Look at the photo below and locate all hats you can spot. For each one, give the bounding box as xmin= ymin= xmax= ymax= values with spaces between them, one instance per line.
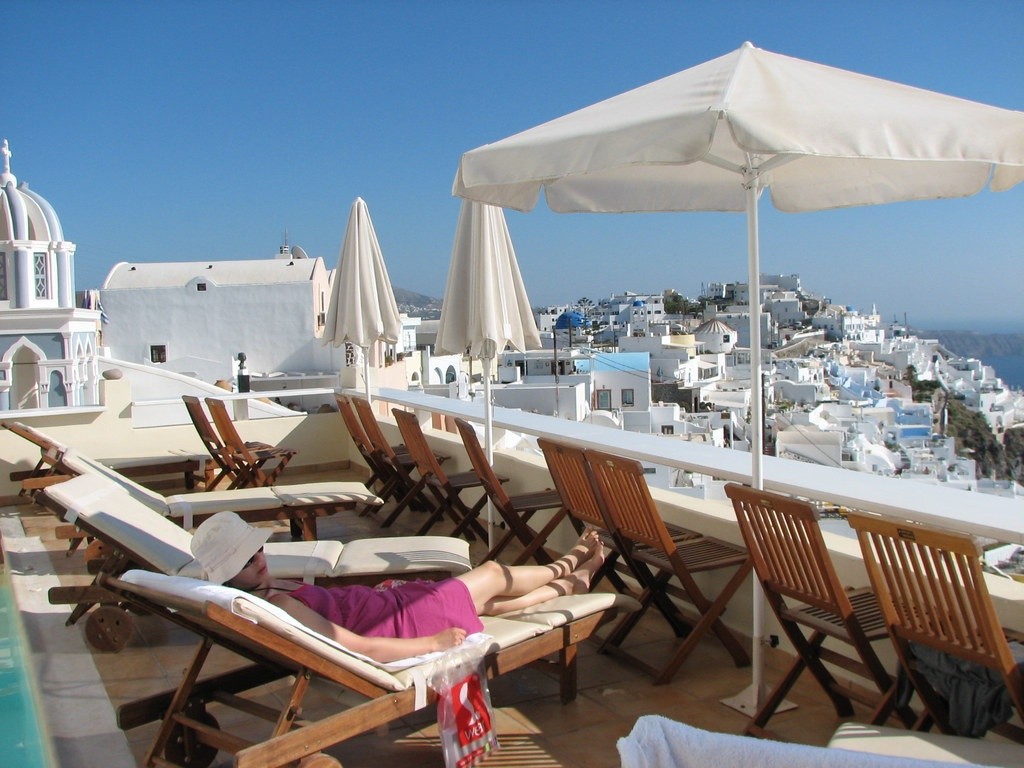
xmin=190 ymin=511 xmax=274 ymax=585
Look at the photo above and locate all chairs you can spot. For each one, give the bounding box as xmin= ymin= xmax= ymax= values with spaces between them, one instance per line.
xmin=0 ymin=387 xmax=1024 ymax=768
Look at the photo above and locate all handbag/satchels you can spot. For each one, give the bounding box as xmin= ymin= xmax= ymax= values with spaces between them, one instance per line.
xmin=423 ymin=646 xmax=500 ymax=768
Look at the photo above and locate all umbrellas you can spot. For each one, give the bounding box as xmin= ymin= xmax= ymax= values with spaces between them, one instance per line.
xmin=450 ymin=40 xmax=1024 ymax=692
xmin=320 ymin=196 xmax=403 ymax=483
xmin=434 ymin=194 xmax=543 ymax=553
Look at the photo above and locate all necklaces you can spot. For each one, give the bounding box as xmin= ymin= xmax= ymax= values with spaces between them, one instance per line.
xmin=251 ymin=585 xmax=302 ymax=597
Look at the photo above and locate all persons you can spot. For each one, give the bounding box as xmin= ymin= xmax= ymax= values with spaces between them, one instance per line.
xmin=187 ymin=510 xmax=608 ymax=664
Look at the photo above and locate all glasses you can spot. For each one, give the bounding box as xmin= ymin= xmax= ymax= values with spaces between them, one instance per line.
xmin=242 ymin=545 xmax=263 ymax=570
